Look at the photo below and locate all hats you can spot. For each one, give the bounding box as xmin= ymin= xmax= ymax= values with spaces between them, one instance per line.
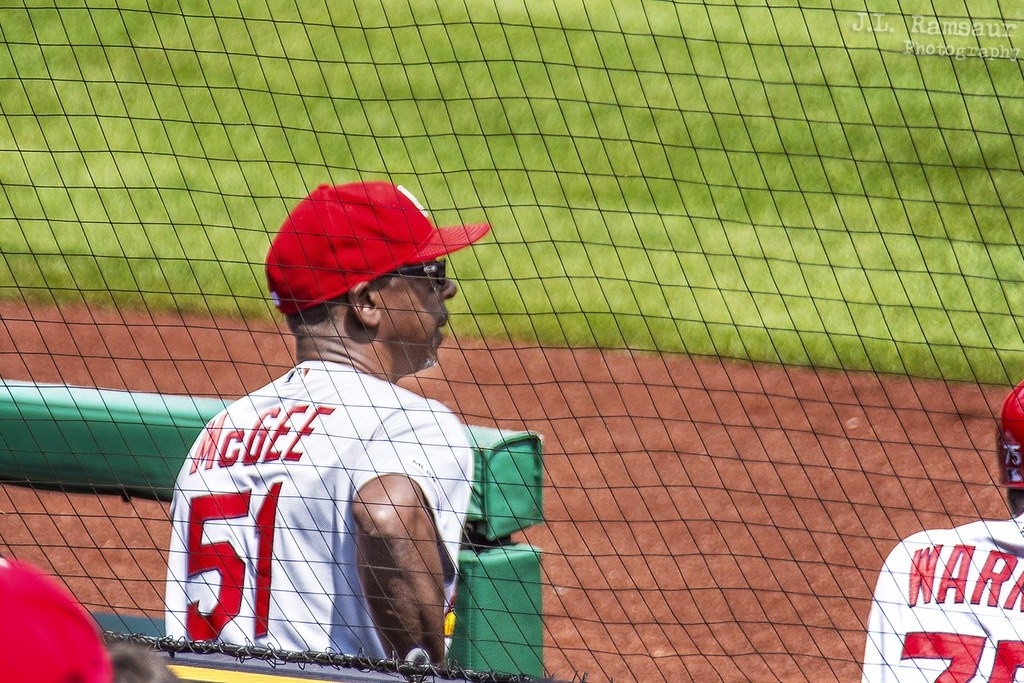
xmin=265 ymin=182 xmax=491 ymax=315
xmin=0 ymin=554 xmax=119 ymax=683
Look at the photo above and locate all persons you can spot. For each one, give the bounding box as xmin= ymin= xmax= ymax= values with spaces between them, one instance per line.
xmin=858 ymin=378 xmax=1024 ymax=683
xmin=162 ymin=182 xmax=476 ymax=672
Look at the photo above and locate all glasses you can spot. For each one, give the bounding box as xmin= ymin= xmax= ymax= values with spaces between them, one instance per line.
xmin=391 ymin=258 xmax=446 ymax=286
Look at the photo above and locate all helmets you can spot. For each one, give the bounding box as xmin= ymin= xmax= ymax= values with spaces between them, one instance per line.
xmin=995 ymin=380 xmax=1024 ymax=488
